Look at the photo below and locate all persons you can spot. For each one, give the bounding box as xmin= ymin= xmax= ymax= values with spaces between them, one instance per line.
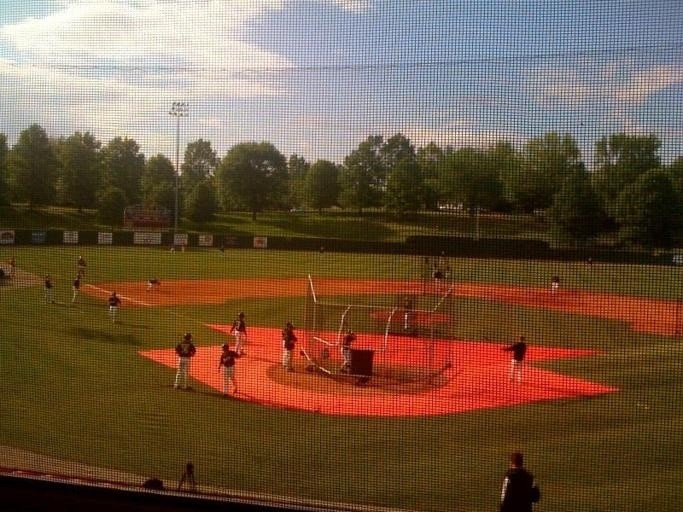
xmin=171 ymin=331 xmax=196 ymax=390
xmin=9 ymin=253 xmax=16 ymax=278
xmin=548 ymin=270 xmax=560 ymax=296
xmin=403 ymin=295 xmax=414 ymax=330
xmin=43 ymin=272 xmax=54 ymax=304
xmin=76 ymin=254 xmax=86 ymax=277
xmin=339 ymin=330 xmax=356 ymax=370
xmin=226 ymin=311 xmax=248 ymax=357
xmin=280 ymin=322 xmax=296 ymax=373
xmin=145 ymin=278 xmax=160 ymax=294
xmin=216 ymin=342 xmax=242 ymax=397
xmin=70 ymin=271 xmax=81 ymax=305
xmin=503 ymin=335 xmax=524 ymax=386
xmin=105 ymin=291 xmax=121 ymax=326
xmin=497 ymin=451 xmax=539 ymax=512
xmin=430 ymin=263 xmax=444 ymax=293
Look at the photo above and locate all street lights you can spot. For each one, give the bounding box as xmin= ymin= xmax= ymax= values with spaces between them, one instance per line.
xmin=169 ymin=101 xmax=190 ymax=228
